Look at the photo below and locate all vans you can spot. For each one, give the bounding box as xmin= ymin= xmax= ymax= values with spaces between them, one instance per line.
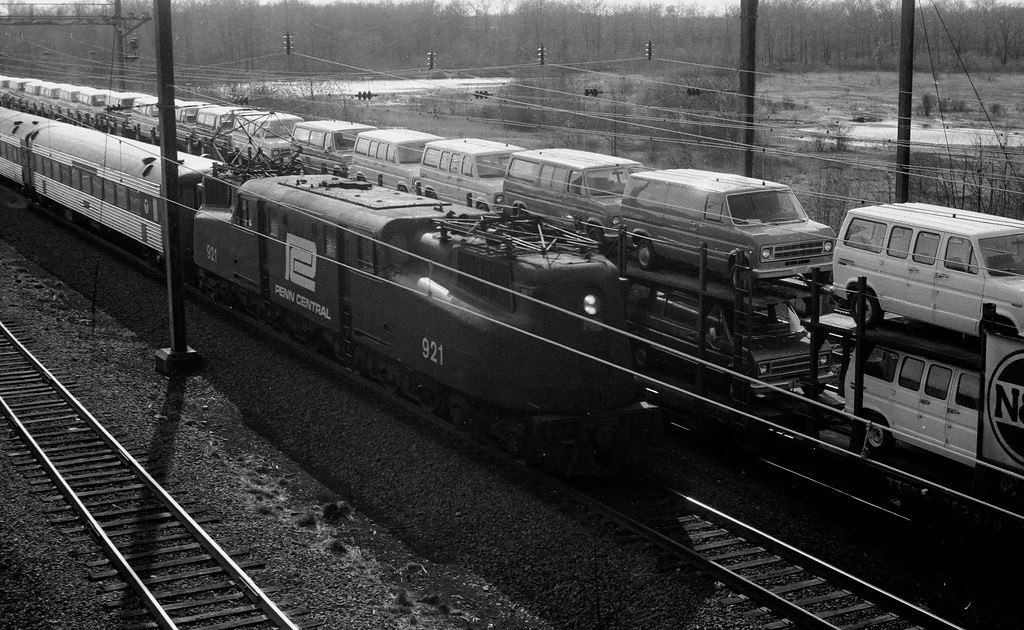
xmin=419 ymin=138 xmax=528 ymax=215
xmin=841 ymin=342 xmax=981 ymax=471
xmin=830 ymin=202 xmax=1024 ymax=343
xmin=193 ymin=105 xmax=252 ymax=156
xmin=618 ymin=168 xmax=837 ymax=298
xmin=130 ymin=97 xmax=184 ymax=145
xmin=230 ymin=110 xmax=305 ymax=169
xmin=289 ymin=118 xmax=379 ymax=177
xmin=0 ymin=75 xmax=155 ymax=137
xmin=349 ymin=127 xmax=446 ymax=196
xmin=174 ymin=100 xmax=213 ymax=154
xmin=502 ymin=148 xmax=647 ymax=250
xmin=623 ymin=280 xmax=835 ymax=402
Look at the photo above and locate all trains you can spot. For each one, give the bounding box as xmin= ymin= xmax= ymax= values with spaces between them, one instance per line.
xmin=0 ymin=106 xmax=664 ymax=484
